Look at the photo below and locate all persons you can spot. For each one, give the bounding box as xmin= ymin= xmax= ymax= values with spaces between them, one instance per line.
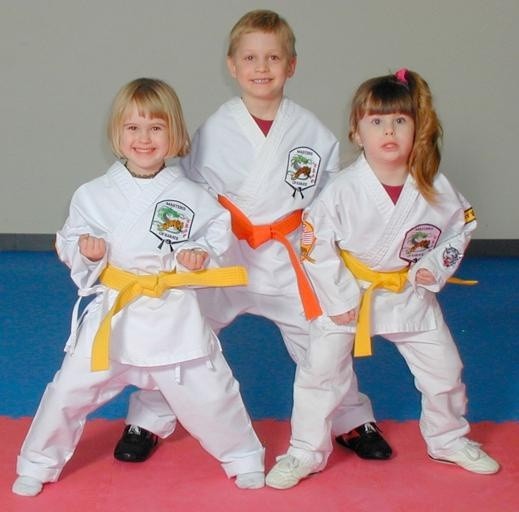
xmin=113 ymin=8 xmax=393 ymax=461
xmin=12 ymin=77 xmax=265 ymax=497
xmin=264 ymin=67 xmax=501 ymax=491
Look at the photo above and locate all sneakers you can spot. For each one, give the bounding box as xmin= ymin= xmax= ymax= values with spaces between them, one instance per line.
xmin=112 ymin=424 xmax=160 ymax=462
xmin=265 ymin=456 xmax=319 ymax=490
xmin=428 ymin=439 xmax=501 ymax=475
xmin=333 ymin=422 xmax=393 ymax=459
